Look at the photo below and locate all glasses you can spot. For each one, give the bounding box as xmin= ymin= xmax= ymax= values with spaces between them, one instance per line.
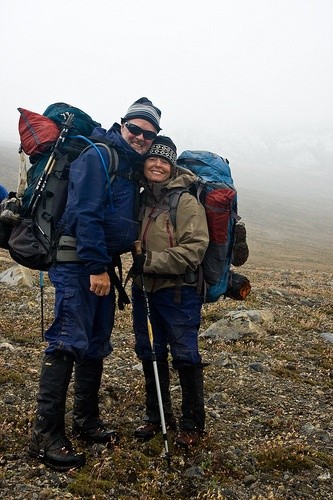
xmin=124 ymin=120 xmax=157 ymax=141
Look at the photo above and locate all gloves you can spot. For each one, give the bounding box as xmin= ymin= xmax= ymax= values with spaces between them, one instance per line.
xmin=134 ymin=254 xmax=146 ymax=267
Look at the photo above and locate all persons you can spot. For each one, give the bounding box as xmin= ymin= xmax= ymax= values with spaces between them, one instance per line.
xmin=133 ymin=135 xmax=210 ymax=446
xmin=26 ymin=97 xmax=161 ymax=471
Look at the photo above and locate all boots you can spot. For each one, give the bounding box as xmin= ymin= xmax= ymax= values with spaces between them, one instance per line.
xmin=174 ymin=363 xmax=204 ymax=447
xmin=71 ymin=356 xmax=120 ymax=445
xmin=133 ymin=361 xmax=174 ymax=438
xmin=27 ymin=354 xmax=85 ymax=471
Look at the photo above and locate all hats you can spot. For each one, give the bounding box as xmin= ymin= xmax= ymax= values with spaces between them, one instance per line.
xmin=124 ymin=96 xmax=161 ymax=131
xmin=143 ymin=135 xmax=177 ymax=169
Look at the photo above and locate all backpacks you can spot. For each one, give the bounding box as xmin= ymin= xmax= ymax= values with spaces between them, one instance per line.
xmin=136 ymin=150 xmax=251 ymax=303
xmin=0 ymin=103 xmax=117 ymax=270
